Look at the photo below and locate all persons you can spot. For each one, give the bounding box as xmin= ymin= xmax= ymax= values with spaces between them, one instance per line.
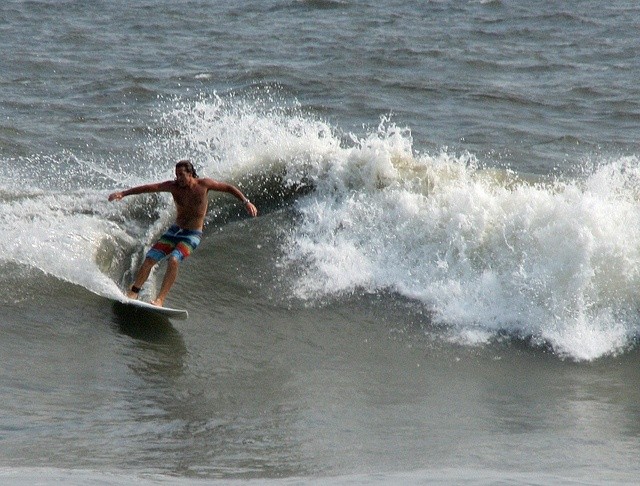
xmin=109 ymin=161 xmax=258 ymax=306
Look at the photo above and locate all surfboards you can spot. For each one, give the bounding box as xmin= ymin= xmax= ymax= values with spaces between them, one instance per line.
xmin=123 ymin=266 xmax=188 ymax=316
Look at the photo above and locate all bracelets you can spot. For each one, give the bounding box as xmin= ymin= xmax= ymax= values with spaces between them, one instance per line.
xmin=243 ymin=199 xmax=250 ymax=205
